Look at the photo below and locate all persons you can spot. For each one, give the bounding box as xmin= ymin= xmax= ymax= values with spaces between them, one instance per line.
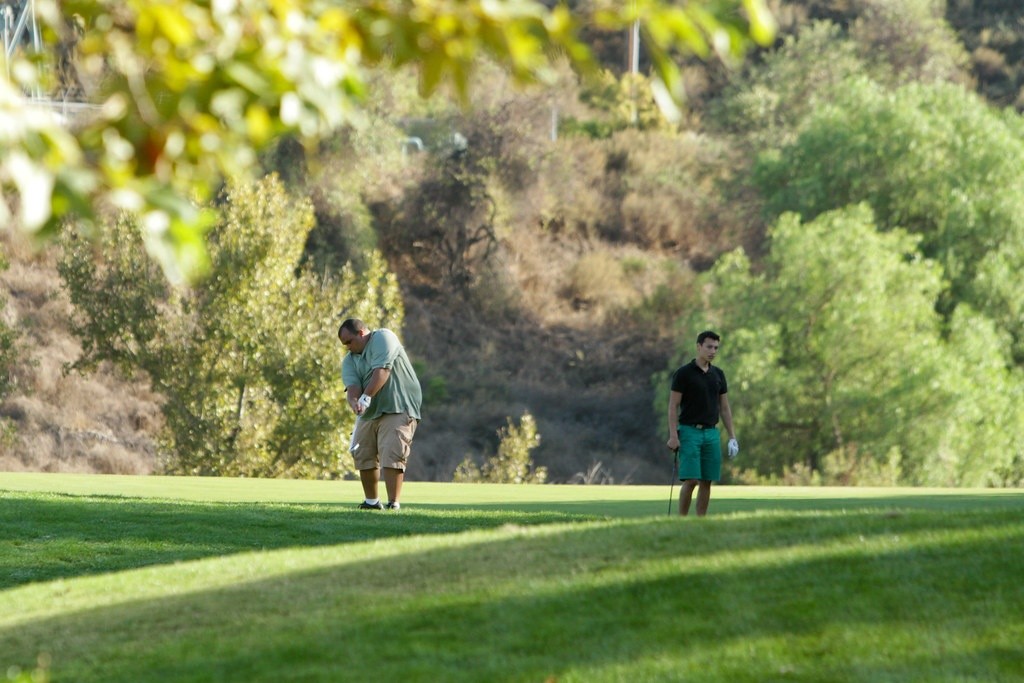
xmin=668 ymin=331 xmax=738 ymax=516
xmin=338 ymin=318 xmax=423 ymax=510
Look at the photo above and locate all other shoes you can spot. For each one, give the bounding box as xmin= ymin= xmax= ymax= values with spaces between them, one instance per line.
xmin=385 ymin=503 xmax=400 ymax=509
xmin=357 ymin=501 xmax=383 ymax=510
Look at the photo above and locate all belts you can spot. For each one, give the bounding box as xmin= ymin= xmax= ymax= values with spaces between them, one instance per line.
xmin=680 ymin=422 xmax=715 ymax=429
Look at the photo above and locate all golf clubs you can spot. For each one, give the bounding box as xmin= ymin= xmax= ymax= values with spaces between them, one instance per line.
xmin=348 ymin=413 xmax=360 ymax=452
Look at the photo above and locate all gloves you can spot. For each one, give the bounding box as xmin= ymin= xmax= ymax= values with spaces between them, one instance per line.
xmin=728 ymin=439 xmax=738 ymax=459
xmin=358 ymin=394 xmax=371 ymax=416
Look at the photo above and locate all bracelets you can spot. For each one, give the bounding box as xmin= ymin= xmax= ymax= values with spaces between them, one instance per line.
xmin=359 ymin=394 xmax=371 ymax=408
